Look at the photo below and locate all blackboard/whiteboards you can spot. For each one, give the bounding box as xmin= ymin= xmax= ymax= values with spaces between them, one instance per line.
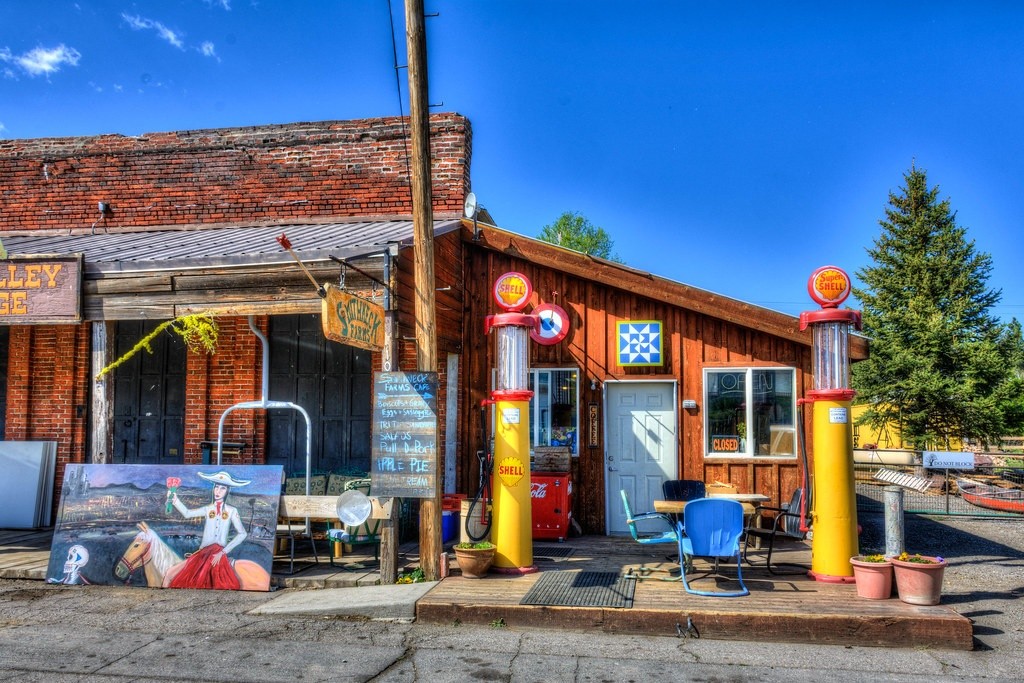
xmin=368 ymin=367 xmax=440 ymax=499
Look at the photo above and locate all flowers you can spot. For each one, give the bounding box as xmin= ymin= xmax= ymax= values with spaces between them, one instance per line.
xmin=860 ymin=555 xmax=886 ymax=563
xmin=898 ymin=552 xmax=944 ymax=564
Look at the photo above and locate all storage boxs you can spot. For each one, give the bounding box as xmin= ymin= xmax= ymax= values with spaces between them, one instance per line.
xmin=704 ymin=483 xmax=740 ymax=494
xmin=418 ymin=510 xmax=459 ymax=544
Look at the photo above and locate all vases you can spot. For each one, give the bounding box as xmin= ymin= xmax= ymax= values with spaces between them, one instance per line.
xmin=890 ymin=555 xmax=947 ymax=606
xmin=850 ymin=556 xmax=894 ymax=599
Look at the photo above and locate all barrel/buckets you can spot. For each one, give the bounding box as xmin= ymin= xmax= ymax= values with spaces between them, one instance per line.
xmin=460 ymin=498 xmax=493 ymax=543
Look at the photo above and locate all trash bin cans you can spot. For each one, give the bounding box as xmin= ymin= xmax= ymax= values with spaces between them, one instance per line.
xmin=460 ymin=497 xmax=491 ymax=544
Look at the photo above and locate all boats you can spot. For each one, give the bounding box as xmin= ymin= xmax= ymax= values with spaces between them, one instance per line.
xmin=955 ymin=479 xmax=1024 ymax=515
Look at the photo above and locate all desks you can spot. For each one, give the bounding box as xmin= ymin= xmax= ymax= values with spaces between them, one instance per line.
xmin=653 ymin=500 xmax=756 ymax=584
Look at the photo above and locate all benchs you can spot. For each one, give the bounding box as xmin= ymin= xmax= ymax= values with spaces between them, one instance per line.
xmin=284 ymin=465 xmax=408 ymax=547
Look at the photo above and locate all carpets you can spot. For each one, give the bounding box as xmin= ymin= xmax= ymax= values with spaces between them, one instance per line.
xmin=517 ymin=569 xmax=638 ymax=609
xmin=532 ymin=546 xmax=576 ymax=568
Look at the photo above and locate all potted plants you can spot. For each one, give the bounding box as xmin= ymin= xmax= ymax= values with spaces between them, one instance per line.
xmin=736 ymin=422 xmax=756 ymax=452
xmin=452 ymin=541 xmax=497 ymax=579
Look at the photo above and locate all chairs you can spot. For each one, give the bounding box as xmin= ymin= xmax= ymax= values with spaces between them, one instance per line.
xmin=620 ymin=489 xmax=690 ymax=582
xmin=662 ymin=480 xmax=729 ymax=564
xmin=272 ymin=495 xmax=319 ymax=575
xmin=326 ymin=479 xmax=382 ymax=572
xmin=677 ymin=498 xmax=750 ymax=598
xmin=744 ymin=487 xmax=813 ymax=577
